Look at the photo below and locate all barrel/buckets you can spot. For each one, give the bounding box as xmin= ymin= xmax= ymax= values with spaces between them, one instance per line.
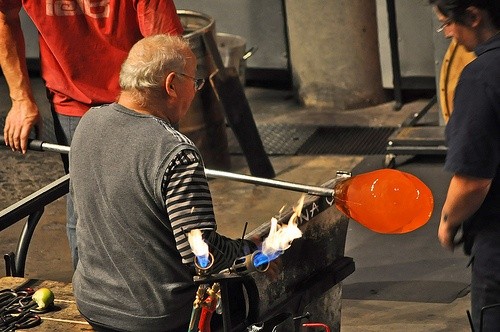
xmin=218 ymin=34 xmax=259 ymax=125
xmin=176 ymin=10 xmax=231 ymax=181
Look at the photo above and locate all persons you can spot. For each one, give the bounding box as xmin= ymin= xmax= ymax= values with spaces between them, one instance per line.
xmin=429 ymin=0 xmax=500 ymax=332
xmin=0 ymin=0 xmax=186 ymax=271
xmin=69 ymin=34 xmax=263 ymax=332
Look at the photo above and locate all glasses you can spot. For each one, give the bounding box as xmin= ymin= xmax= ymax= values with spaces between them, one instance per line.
xmin=181 ymin=73 xmax=205 ymax=90
xmin=432 ymin=17 xmax=453 ymax=36
